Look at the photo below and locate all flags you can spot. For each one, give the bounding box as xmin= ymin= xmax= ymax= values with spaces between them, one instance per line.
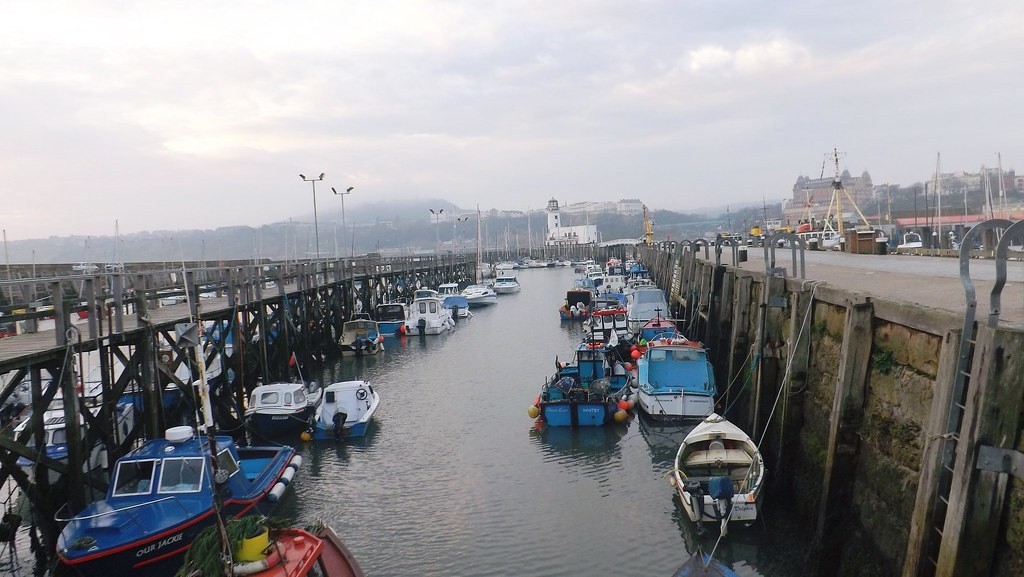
xmin=289 ymin=355 xmax=295 ymax=366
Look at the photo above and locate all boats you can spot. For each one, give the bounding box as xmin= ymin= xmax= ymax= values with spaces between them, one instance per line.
xmin=1 ymin=257 xmax=765 ymax=577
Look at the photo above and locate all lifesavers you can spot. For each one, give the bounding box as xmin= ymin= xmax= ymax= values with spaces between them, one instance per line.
xmin=586 ymin=342 xmax=604 ymax=349
xmin=225 ymin=539 xmax=285 ymax=575
